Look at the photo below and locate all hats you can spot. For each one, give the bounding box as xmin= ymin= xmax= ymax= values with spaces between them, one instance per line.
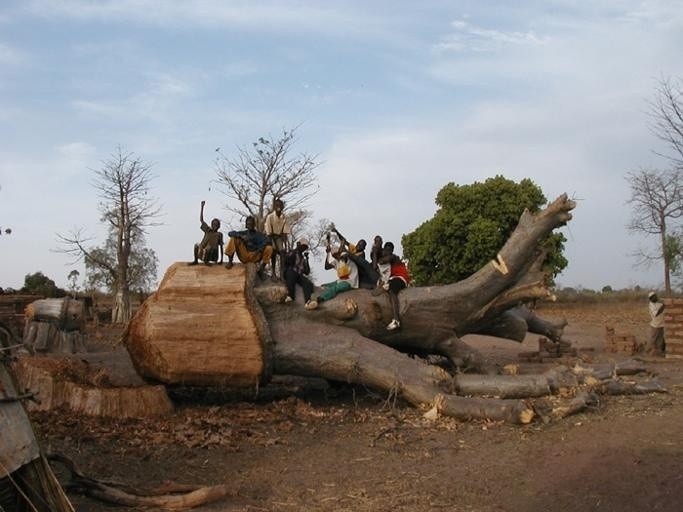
xmin=300 ymin=238 xmax=309 ymax=248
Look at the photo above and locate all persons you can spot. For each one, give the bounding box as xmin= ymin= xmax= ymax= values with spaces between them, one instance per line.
xmin=223 ymin=215 xmax=273 ymax=281
xmin=281 ymin=237 xmax=318 ymax=310
xmin=641 ymin=290 xmax=667 ymax=359
xmin=263 ymin=199 xmax=289 ymax=282
xmin=313 ymin=221 xmax=410 ymax=330
xmin=186 ymin=200 xmax=224 ymax=267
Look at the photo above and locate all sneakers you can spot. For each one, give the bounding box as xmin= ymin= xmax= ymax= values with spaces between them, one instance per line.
xmin=339 ymin=239 xmax=346 ymax=253
xmin=304 ymin=296 xmax=322 ymax=310
xmin=285 ymin=296 xmax=292 ymax=303
xmin=386 ymin=318 xmax=400 ymax=330
xmin=382 ymin=283 xmax=392 ymax=291
